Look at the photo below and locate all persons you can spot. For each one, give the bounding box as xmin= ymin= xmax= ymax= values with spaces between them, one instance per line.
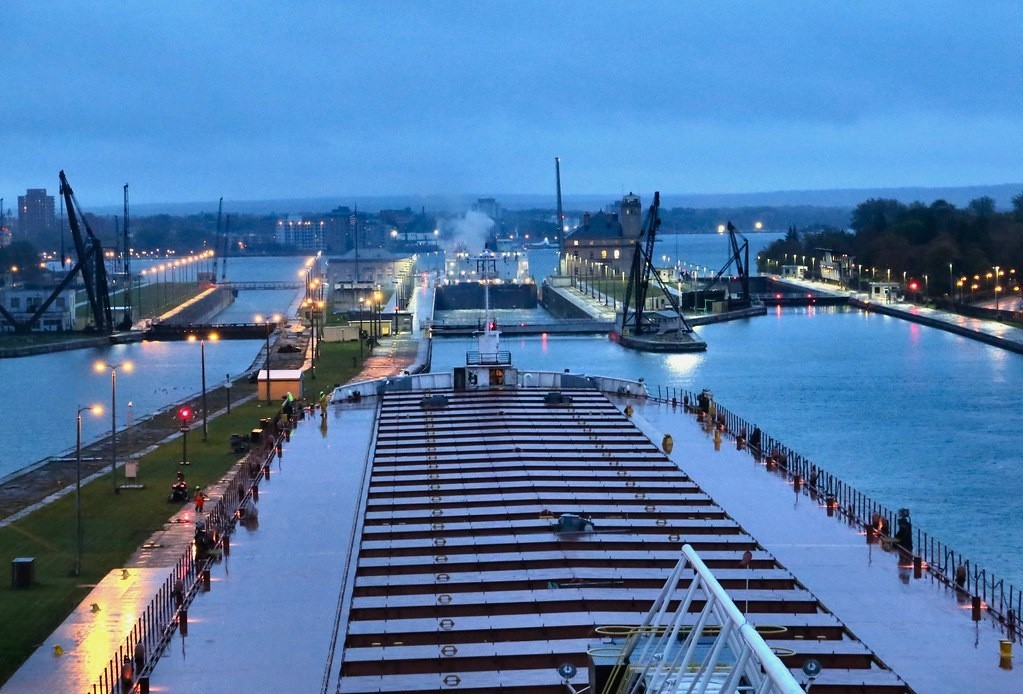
xmin=262 ymin=430 xmax=274 ymax=451
xmin=281 ymin=395 xmax=292 ymax=419
xmin=237 ymin=483 xmax=246 ymax=502
xmin=251 ymin=455 xmax=260 ymax=477
xmin=195 ymin=486 xmax=209 ymax=512
xmin=319 ymin=391 xmax=327 ymax=416
xmin=176 ymin=471 xmax=184 ymax=481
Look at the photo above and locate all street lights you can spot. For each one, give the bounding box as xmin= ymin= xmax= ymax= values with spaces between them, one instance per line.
xmin=857 ymin=264 xmax=863 ymax=292
xmin=358 ymin=297 xmax=365 ymax=357
xmin=921 ymin=274 xmax=929 ymax=307
xmin=136 ymin=248 xmax=216 ymax=324
xmin=297 ymin=255 xmax=327 ymax=379
xmin=366 ymin=299 xmax=374 ymax=351
xmin=254 ymin=313 xmax=282 ymax=405
xmin=872 ymin=267 xmax=876 ymax=282
xmin=375 ymin=293 xmax=384 ymax=338
xmin=886 ymin=268 xmax=892 ymax=305
xmin=188 ymin=332 xmax=220 ymax=442
xmin=811 ymin=257 xmax=815 ymax=280
xmin=76 ymin=406 xmax=104 ymax=575
xmin=802 ymin=256 xmax=805 ymax=280
xmin=960 ymin=276 xmax=967 ymax=305
xmin=992 ymin=265 xmax=1000 ymax=310
xmin=94 ymin=360 xmax=135 ymax=493
xmin=784 ymin=253 xmax=787 ymax=277
xmin=793 ymin=254 xmax=797 ymax=279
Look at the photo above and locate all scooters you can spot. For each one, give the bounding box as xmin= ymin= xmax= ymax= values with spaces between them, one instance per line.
xmin=168 ymin=482 xmax=190 ymax=502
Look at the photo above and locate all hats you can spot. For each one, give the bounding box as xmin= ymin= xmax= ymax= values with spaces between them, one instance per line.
xmin=196 ymin=486 xmax=200 ymax=489
xmin=320 ymin=391 xmax=323 ymax=395
xmin=287 ymin=392 xmax=290 ymax=394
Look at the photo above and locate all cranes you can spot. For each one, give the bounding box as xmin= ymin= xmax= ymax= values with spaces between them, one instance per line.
xmin=617 ymin=190 xmax=694 ymax=336
xmin=700 ymin=219 xmax=752 ymax=312
xmin=115 ymin=182 xmax=134 ymax=327
xmin=211 ymin=194 xmax=230 ymax=287
xmin=55 ymin=168 xmax=115 ymax=334
xmin=552 ymin=156 xmax=566 ymax=275
